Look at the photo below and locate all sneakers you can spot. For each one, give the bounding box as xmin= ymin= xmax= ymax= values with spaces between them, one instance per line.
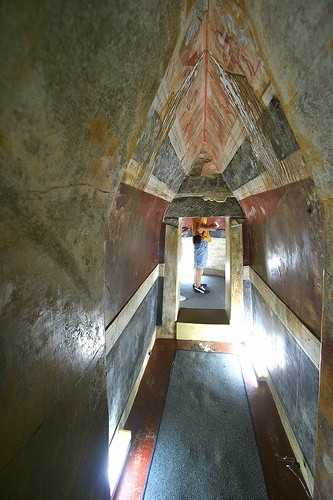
xmin=194 ymin=286 xmax=210 ymax=294
xmin=193 ymin=284 xmax=207 ymax=289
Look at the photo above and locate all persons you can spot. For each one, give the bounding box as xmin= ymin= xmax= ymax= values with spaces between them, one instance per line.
xmin=191 ymin=217 xmax=218 ymax=295
